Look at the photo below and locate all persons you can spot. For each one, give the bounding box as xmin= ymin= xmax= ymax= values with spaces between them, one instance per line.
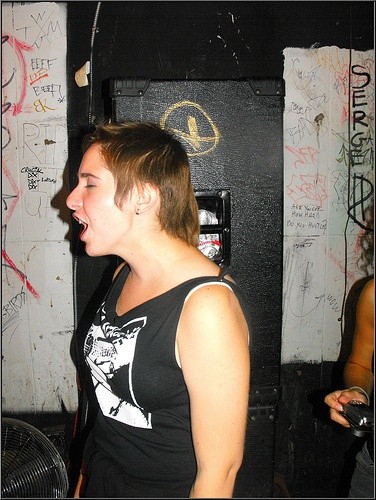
xmin=66 ymin=123 xmax=249 ymax=499
xmin=325 ymin=279 xmax=375 ymax=498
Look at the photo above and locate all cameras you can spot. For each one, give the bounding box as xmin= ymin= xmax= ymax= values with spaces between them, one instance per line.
xmin=338 ymin=400 xmax=374 ymax=437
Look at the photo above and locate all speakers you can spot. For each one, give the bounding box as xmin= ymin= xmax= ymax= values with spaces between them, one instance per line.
xmin=105 ymin=77 xmax=285 ymax=500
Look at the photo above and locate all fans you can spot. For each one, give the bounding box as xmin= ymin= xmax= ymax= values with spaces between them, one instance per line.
xmin=2 ymin=417 xmax=69 ymax=498
